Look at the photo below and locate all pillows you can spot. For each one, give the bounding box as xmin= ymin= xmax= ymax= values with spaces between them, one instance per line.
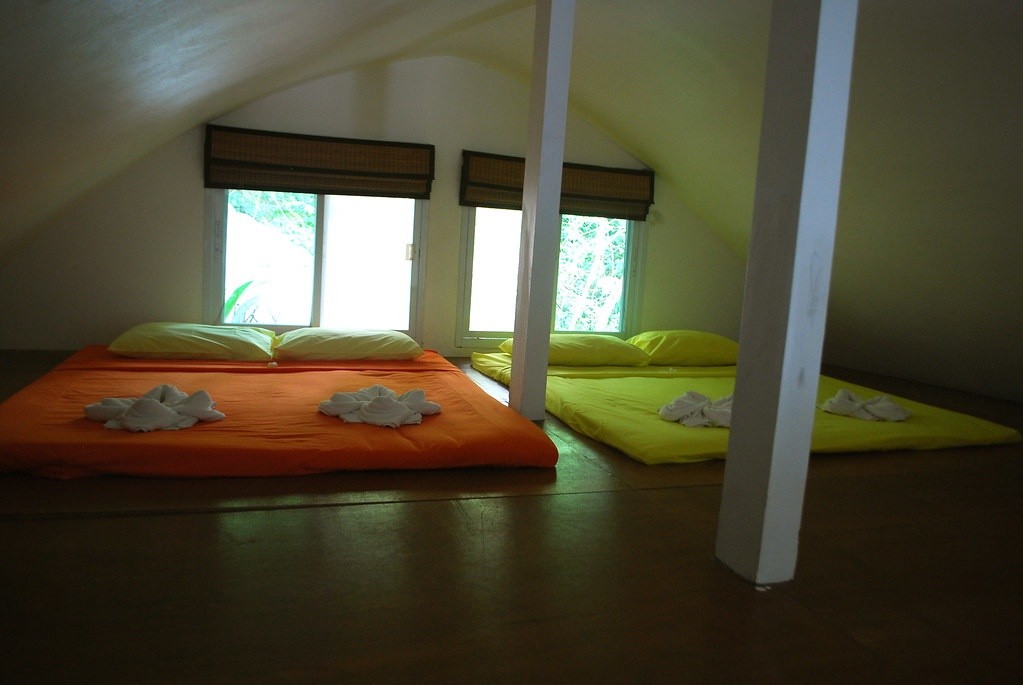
xmin=626 ymin=330 xmax=740 ymax=367
xmin=274 ymin=328 xmax=426 ymax=362
xmin=106 ymin=321 xmax=275 ymax=363
xmin=502 ymin=332 xmax=653 ymax=368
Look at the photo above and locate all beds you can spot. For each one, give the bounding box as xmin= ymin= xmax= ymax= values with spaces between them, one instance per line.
xmin=0 ymin=341 xmax=559 ymax=480
xmin=471 ymin=330 xmax=1023 ymax=465
